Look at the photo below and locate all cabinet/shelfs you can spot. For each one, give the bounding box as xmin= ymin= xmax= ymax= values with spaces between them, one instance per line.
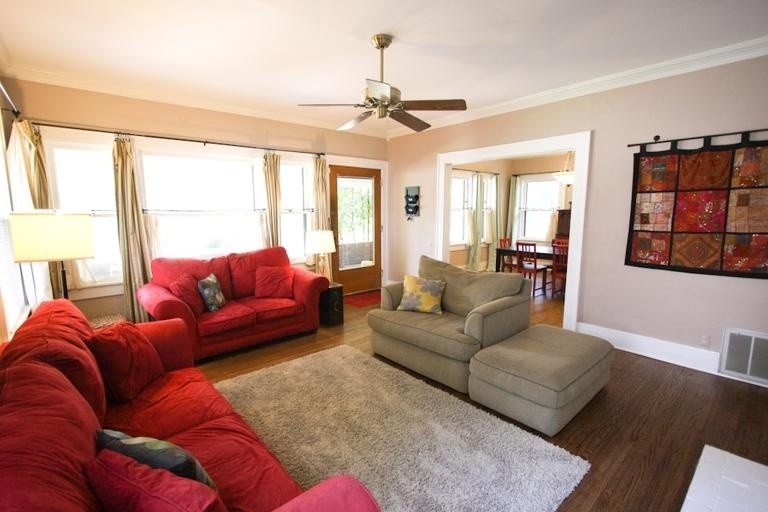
xmin=556 ymin=210 xmax=570 ymax=240
xmin=320 ymin=282 xmax=344 ymax=328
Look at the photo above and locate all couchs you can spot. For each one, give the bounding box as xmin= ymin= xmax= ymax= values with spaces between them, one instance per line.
xmin=0 ymin=297 xmax=382 ymax=512
xmin=136 ymin=247 xmax=329 ymax=366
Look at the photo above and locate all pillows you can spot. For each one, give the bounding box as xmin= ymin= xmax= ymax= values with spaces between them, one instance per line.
xmin=254 ymin=266 xmax=294 ymax=297
xmin=395 ymin=275 xmax=447 ymax=314
xmin=95 ymin=429 xmax=218 ymax=492
xmin=83 ymin=321 xmax=164 ymax=404
xmin=86 ymin=448 xmax=228 ymax=512
xmin=197 ymin=272 xmax=226 ymax=312
xmin=169 ymin=273 xmax=208 ymax=318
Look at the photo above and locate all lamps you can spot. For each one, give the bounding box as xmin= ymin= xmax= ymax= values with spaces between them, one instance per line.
xmin=9 ymin=210 xmax=94 ymax=298
xmin=305 ymin=230 xmax=336 ymax=284
xmin=552 ymin=151 xmax=574 ymax=187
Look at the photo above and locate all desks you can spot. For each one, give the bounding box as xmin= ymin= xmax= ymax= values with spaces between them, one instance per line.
xmin=90 ymin=312 xmax=127 ymax=326
xmin=496 ymin=245 xmax=568 ymax=272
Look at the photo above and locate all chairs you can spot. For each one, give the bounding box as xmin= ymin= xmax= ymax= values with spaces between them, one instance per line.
xmin=366 ymin=253 xmax=532 ymax=395
xmin=543 ymin=240 xmax=569 ymax=296
xmin=551 ymin=239 xmax=569 ymax=299
xmin=499 ymin=238 xmax=517 ymax=273
xmin=514 ymin=242 xmax=548 ymax=301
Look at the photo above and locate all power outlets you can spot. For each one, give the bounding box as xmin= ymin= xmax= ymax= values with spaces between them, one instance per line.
xmin=700 ymin=334 xmax=709 ymax=346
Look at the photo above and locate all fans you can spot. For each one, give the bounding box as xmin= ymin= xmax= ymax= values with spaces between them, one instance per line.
xmin=297 ymin=32 xmax=467 ymax=133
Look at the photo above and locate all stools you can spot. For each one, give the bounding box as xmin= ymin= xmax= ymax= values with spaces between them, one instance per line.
xmin=468 ymin=323 xmax=615 ymax=437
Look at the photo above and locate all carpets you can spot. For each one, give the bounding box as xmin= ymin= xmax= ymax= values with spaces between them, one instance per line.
xmin=213 ymin=344 xmax=592 ymax=511
